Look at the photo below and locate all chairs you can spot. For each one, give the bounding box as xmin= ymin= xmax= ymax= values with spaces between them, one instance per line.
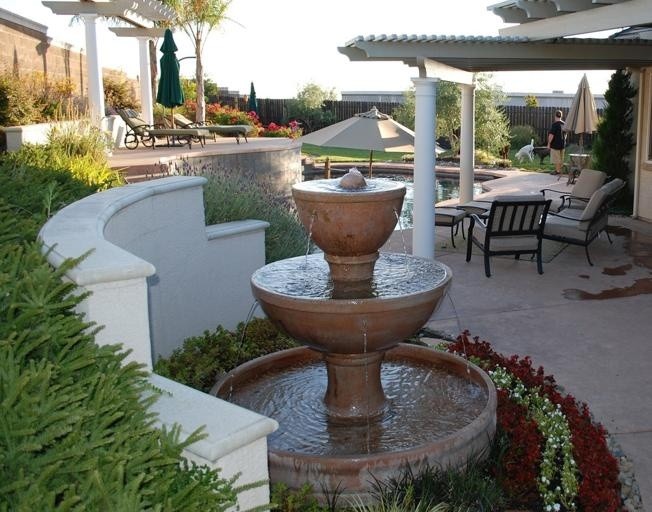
xmin=466 ymin=168 xmax=627 ymax=279
xmin=112 ymin=105 xmax=252 ymax=149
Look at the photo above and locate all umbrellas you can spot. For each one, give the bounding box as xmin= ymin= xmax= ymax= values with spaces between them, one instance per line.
xmin=156 ymin=28 xmax=185 ymax=145
xmin=562 ymin=73 xmax=598 ymax=154
xmin=300 ymin=106 xmax=447 ymax=178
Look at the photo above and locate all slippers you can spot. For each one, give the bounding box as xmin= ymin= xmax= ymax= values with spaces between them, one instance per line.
xmin=550 ymin=170 xmax=561 ymax=175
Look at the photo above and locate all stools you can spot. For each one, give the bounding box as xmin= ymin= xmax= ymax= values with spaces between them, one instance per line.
xmin=557 ymin=162 xmax=572 ymax=181
xmin=455 ymin=202 xmax=492 ymax=240
xmin=435 ymin=207 xmax=467 ymax=248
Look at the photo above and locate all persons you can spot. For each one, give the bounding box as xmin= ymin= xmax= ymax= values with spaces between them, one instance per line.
xmin=547 ymin=110 xmax=567 ymax=177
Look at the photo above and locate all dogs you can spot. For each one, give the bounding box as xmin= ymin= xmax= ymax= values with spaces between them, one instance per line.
xmin=515 ymin=138 xmax=534 ymax=165
xmin=533 ymin=147 xmax=550 ymax=164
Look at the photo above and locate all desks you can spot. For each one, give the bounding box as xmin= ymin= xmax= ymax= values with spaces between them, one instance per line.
xmin=567 ymin=153 xmax=591 ymax=186
